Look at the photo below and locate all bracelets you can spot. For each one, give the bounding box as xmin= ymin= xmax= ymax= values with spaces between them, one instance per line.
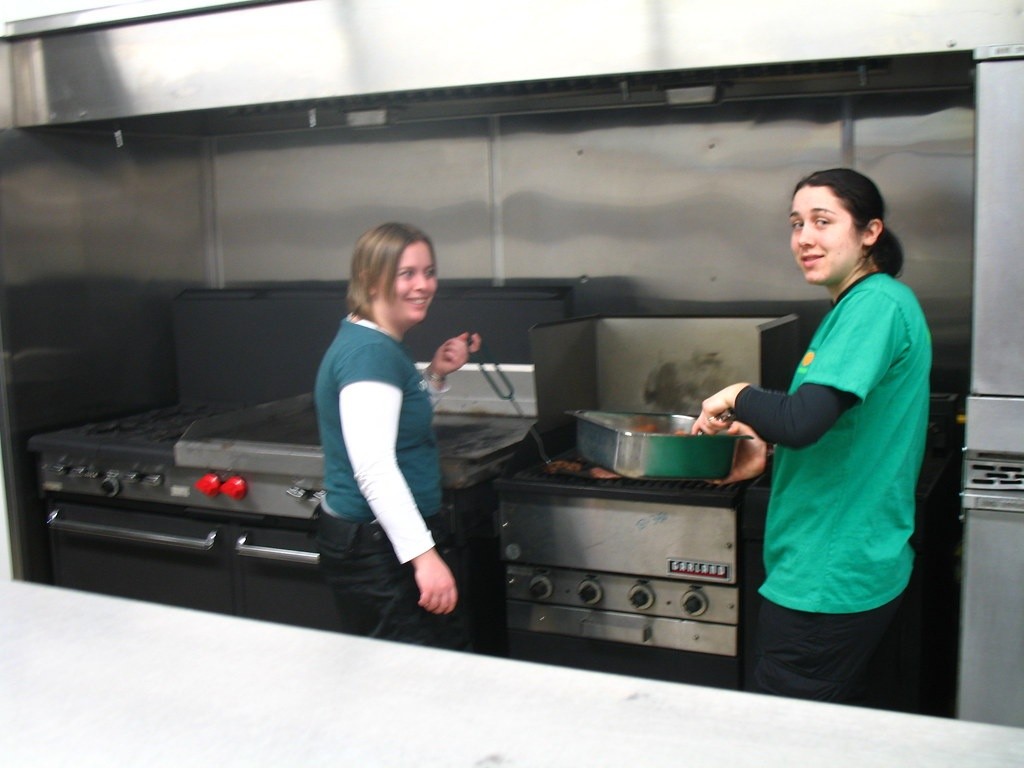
xmin=423 ymin=369 xmax=447 ymax=383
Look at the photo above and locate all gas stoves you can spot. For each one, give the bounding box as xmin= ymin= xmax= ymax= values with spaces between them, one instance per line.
xmin=28 ymin=401 xmax=324 ymax=520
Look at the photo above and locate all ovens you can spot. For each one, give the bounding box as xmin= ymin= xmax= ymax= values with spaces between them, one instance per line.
xmin=47 ymin=503 xmax=344 ymax=634
xmin=505 ymin=562 xmax=739 ymax=691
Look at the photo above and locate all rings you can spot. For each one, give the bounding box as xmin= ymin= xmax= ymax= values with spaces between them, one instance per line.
xmin=709 ymin=415 xmax=716 ymax=422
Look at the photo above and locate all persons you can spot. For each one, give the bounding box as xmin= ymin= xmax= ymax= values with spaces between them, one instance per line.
xmin=692 ymin=168 xmax=933 ymax=703
xmin=315 ymin=222 xmax=481 ymax=645
